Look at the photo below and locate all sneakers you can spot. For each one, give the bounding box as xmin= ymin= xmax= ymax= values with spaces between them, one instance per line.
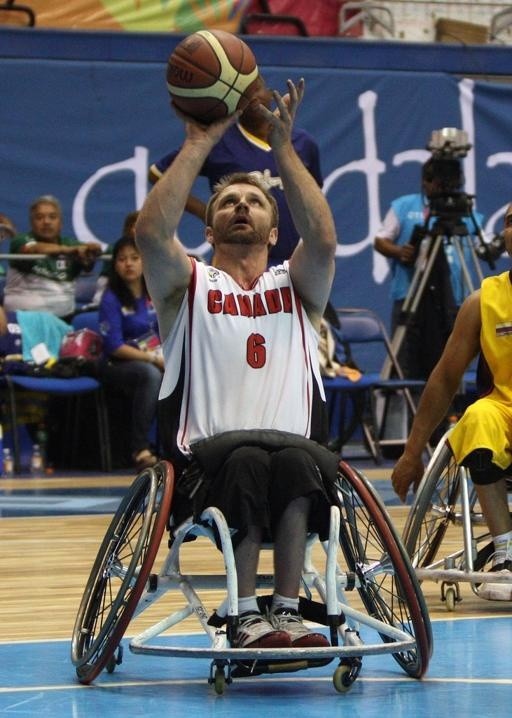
xmin=232 ymin=611 xmax=291 ymax=665
xmin=269 ymin=607 xmax=330 ymax=660
xmin=128 ymin=447 xmax=156 ymax=469
xmin=479 ymin=540 xmax=512 ymax=601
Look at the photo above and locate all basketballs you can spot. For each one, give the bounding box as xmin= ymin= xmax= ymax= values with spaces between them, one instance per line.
xmin=166 ymin=29 xmax=258 ymax=122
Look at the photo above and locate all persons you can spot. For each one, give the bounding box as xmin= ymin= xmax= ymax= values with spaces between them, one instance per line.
xmin=367 ymin=152 xmax=483 ymax=379
xmin=1 ymin=184 xmax=347 ymax=487
xmin=127 ymin=75 xmax=347 ymax=669
xmin=142 ymin=73 xmax=322 ymax=279
xmin=388 ymin=198 xmax=511 ymax=609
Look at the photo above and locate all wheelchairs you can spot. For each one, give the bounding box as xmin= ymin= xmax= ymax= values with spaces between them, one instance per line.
xmin=69 ymin=430 xmax=434 ymax=693
xmin=404 ymin=417 xmax=512 ymax=611
